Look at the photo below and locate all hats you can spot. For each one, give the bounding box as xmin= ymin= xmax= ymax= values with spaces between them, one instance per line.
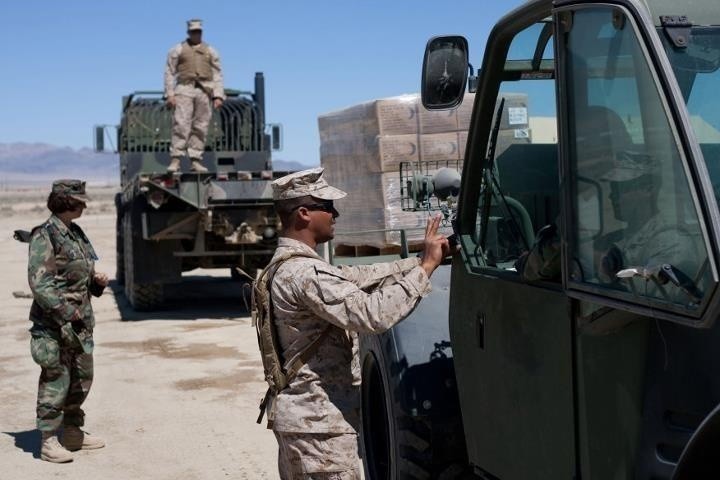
xmin=187 ymin=20 xmax=202 ymax=31
xmin=271 ymin=168 xmax=348 ymax=200
xmin=599 ymin=149 xmax=661 ymax=182
xmin=61 ymin=320 xmax=94 ymax=355
xmin=52 ymin=180 xmax=93 ymax=202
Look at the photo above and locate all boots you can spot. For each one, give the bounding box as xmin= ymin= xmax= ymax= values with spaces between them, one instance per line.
xmin=190 ymin=161 xmax=208 ymax=172
xmin=167 ymin=158 xmax=181 ymax=172
xmin=40 ymin=430 xmax=74 ymax=463
xmin=61 ymin=427 xmax=104 ymax=450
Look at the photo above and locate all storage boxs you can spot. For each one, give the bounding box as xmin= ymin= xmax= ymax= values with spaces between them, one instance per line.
xmin=317 ymin=92 xmax=530 ymax=246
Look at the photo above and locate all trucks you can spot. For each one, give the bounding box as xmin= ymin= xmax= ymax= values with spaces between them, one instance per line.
xmin=328 ymin=0 xmax=720 ymax=480
xmin=93 ymin=70 xmax=292 ymax=313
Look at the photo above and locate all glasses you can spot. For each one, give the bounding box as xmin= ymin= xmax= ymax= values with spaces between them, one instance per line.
xmin=289 ymin=202 xmax=334 ymax=214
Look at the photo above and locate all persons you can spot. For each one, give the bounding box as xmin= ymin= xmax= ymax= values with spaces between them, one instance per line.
xmin=574 ymin=150 xmax=699 ymax=304
xmin=160 ymin=17 xmax=226 ymax=173
xmin=241 ymin=164 xmax=464 ymax=480
xmin=12 ymin=177 xmax=111 ymax=463
xmin=511 ymin=104 xmax=636 ymax=285
